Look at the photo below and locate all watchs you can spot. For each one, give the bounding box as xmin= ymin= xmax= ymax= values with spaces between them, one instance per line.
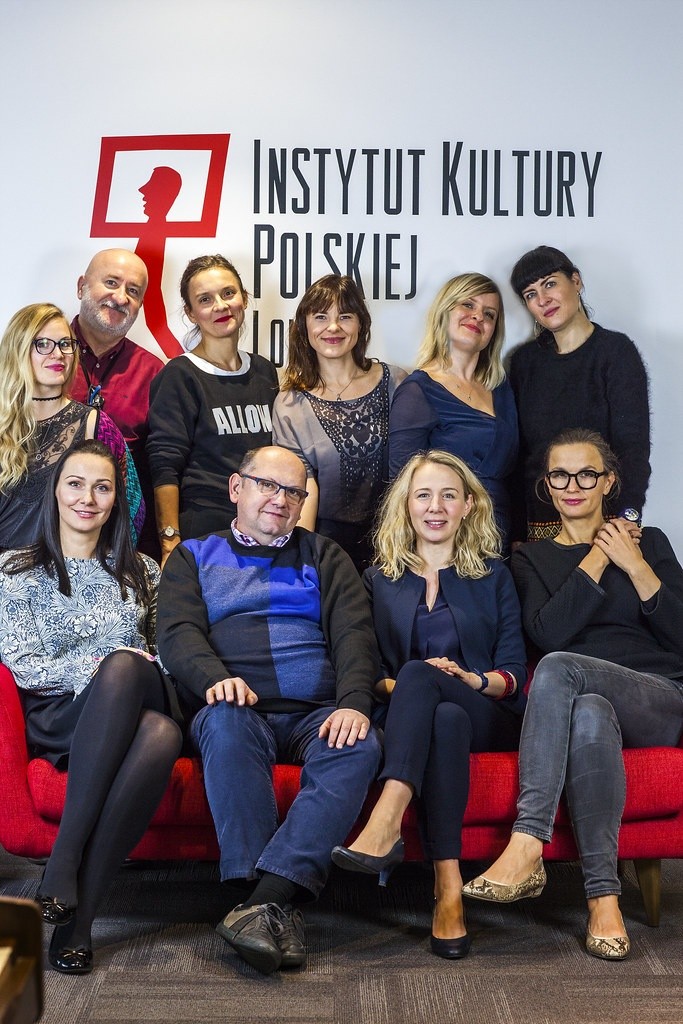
xmin=618 ymin=507 xmax=641 ymax=523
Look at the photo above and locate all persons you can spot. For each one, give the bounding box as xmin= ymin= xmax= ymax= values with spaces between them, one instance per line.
xmin=388 ymin=271 xmax=521 ymax=539
xmin=0 ymin=302 xmax=147 ymax=552
xmin=64 ymin=248 xmax=166 ymax=450
xmin=154 ymin=446 xmax=386 ymax=969
xmin=144 ymin=255 xmax=281 ymax=570
xmin=331 ymin=449 xmax=528 ymax=958
xmin=461 ymin=428 xmax=683 ymax=958
xmin=501 ymin=244 xmax=653 ymax=546
xmin=0 ymin=440 xmax=182 ymax=976
xmin=272 ymin=273 xmax=408 ymax=577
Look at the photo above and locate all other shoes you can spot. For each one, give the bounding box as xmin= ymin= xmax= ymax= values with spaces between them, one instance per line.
xmin=462 ymin=859 xmax=547 ymax=903
xmin=587 ymin=907 xmax=630 ymax=960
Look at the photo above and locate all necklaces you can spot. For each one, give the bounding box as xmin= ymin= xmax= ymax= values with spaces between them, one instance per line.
xmin=326 ymin=368 xmax=357 ymax=401
xmin=32 ymin=393 xmax=62 ymax=401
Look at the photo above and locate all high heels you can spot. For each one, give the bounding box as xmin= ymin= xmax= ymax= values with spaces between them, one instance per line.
xmin=330 ymin=836 xmax=405 ymax=887
xmin=430 ymin=894 xmax=470 ymax=958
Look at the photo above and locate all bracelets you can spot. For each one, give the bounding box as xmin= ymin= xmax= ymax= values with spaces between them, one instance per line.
xmin=492 ymin=669 xmax=514 ymax=700
xmin=471 ymin=667 xmax=489 ymax=692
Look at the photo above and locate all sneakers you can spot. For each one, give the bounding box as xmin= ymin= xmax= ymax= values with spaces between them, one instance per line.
xmin=273 ymin=902 xmax=306 ymax=965
xmin=216 ymin=899 xmax=289 ymax=972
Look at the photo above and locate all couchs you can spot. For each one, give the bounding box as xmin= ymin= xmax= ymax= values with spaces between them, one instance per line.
xmin=0 ymin=661 xmax=683 ymax=930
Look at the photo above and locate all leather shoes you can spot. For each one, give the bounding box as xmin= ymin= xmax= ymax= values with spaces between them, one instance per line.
xmin=34 ymin=861 xmax=80 ymax=926
xmin=48 ymin=921 xmax=92 ymax=973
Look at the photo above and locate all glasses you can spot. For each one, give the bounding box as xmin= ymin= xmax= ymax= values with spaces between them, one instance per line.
xmin=241 ymin=472 xmax=309 ymax=506
xmin=33 ymin=337 xmax=79 ymax=354
xmin=545 ymin=469 xmax=609 ymax=490
